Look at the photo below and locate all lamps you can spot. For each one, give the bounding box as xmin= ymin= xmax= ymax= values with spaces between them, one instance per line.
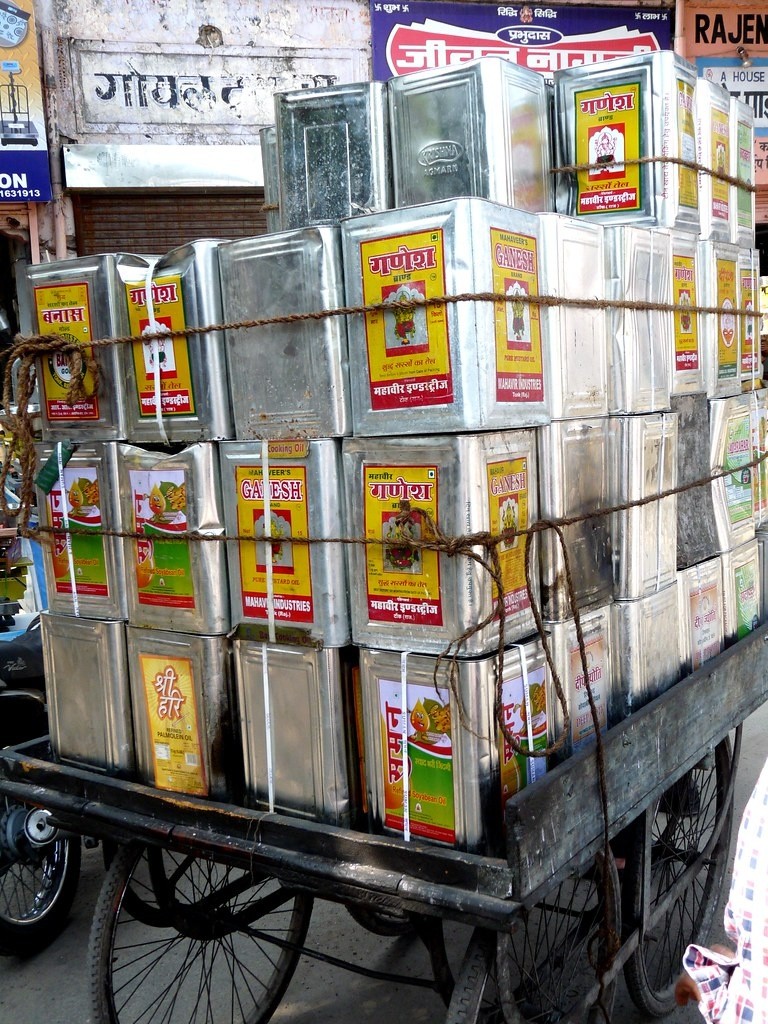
xmin=736 ymin=46 xmax=753 ymax=68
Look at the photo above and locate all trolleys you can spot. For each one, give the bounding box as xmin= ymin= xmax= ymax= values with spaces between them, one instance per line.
xmin=0 ymin=620 xmax=768 ymax=1024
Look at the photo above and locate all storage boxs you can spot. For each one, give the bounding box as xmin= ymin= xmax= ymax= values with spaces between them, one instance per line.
xmin=22 ymin=48 xmax=768 ymax=853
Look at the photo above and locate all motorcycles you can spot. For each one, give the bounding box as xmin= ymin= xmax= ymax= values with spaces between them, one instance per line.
xmin=0 ymin=613 xmax=82 ymax=958
